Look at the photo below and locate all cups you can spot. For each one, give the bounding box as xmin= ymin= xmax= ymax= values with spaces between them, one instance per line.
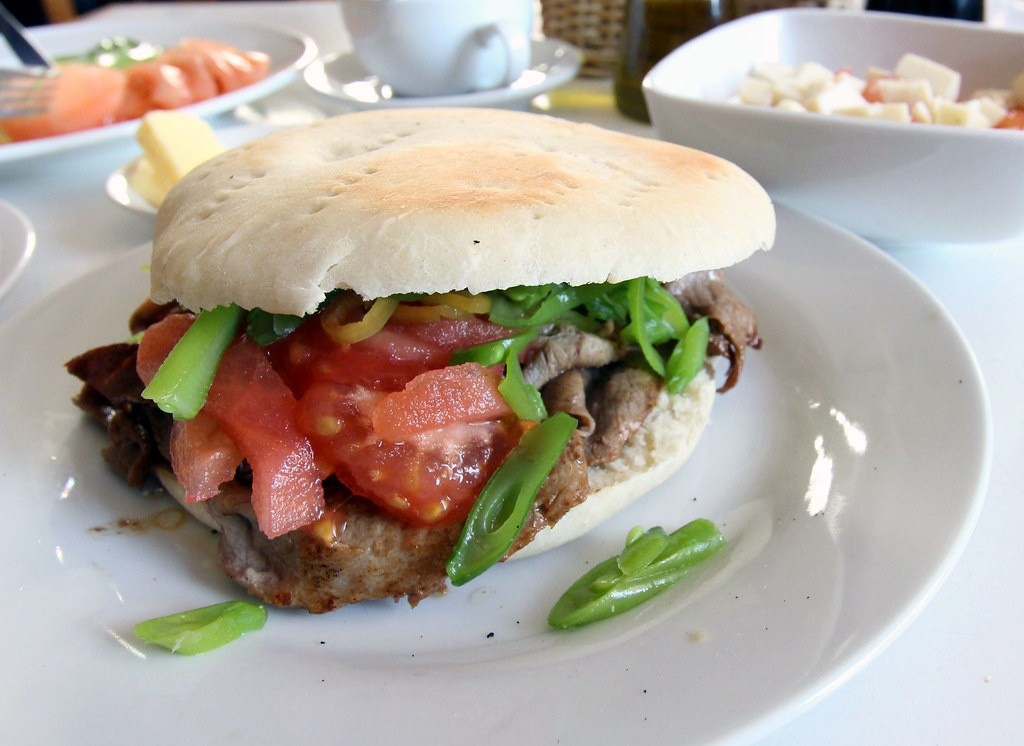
xmin=340 ymin=0 xmax=534 ymax=95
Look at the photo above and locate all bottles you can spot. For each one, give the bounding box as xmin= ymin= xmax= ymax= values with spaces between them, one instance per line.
xmin=614 ymin=0 xmax=742 ymax=127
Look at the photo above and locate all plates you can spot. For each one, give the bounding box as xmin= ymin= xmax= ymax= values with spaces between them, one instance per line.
xmin=0 ymin=199 xmax=993 ymax=746
xmin=0 ymin=199 xmax=36 ymax=300
xmin=107 ymin=126 xmax=261 ymax=216
xmin=0 ymin=18 xmax=319 ymax=172
xmin=304 ymin=37 xmax=584 ymax=111
xmin=641 ymin=7 xmax=1024 ymax=243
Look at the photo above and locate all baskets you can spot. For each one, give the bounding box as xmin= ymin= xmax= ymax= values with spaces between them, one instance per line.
xmin=540 ymin=0 xmax=629 ymax=78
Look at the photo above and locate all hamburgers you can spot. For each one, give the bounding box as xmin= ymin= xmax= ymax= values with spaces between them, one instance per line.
xmin=65 ymin=101 xmax=776 ymax=611
